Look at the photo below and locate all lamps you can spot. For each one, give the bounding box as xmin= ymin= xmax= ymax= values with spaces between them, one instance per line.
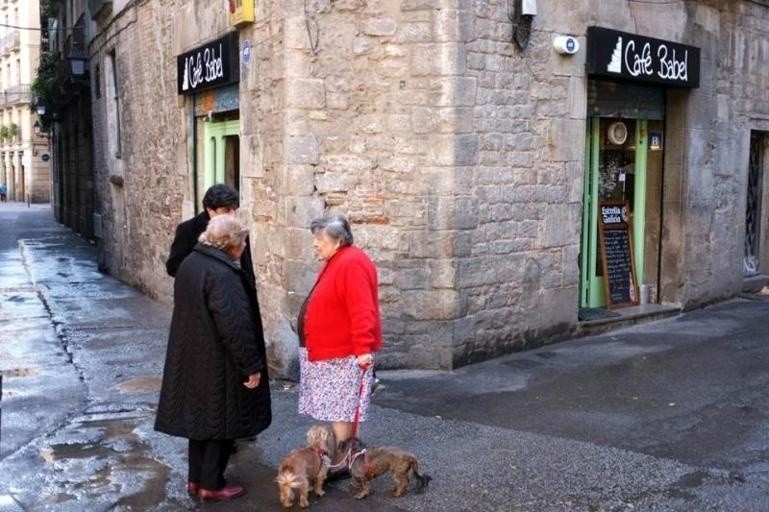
xmin=34 ymin=95 xmax=58 ymax=120
xmin=64 ymin=26 xmax=100 ymax=77
xmin=33 ymin=120 xmax=52 ymax=140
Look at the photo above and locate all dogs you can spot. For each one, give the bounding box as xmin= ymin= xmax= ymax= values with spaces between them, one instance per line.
xmin=272 ymin=424 xmax=336 ymax=508
xmin=337 ymin=437 xmax=433 ymax=502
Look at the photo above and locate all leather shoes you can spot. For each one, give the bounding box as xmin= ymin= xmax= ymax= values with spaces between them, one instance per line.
xmin=323 ymin=466 xmax=351 ymax=484
xmin=188 ymin=482 xmax=244 ymax=505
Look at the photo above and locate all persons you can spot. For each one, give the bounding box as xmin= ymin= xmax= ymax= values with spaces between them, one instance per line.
xmin=166 ymin=184 xmax=259 ymax=455
xmin=152 ymin=213 xmax=272 ymax=501
xmin=298 ymin=215 xmax=382 ymax=484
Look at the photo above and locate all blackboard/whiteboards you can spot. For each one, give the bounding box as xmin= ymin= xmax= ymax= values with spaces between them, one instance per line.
xmin=601 ymin=201 xmax=640 ymax=309
